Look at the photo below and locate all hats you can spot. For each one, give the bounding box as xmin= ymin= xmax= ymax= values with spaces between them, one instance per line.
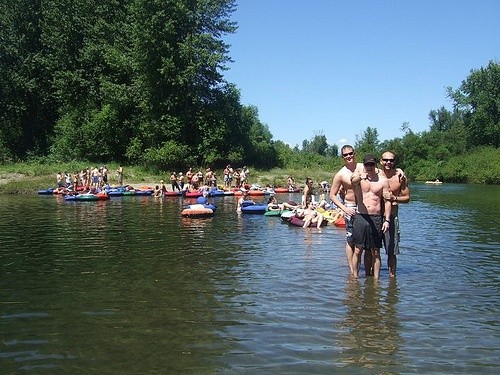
xmin=363 ymin=155 xmax=376 ymax=165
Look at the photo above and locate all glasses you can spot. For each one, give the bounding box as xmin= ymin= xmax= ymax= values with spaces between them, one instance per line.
xmin=382 ymin=158 xmax=395 ymax=162
xmin=342 ymin=152 xmax=354 ymax=157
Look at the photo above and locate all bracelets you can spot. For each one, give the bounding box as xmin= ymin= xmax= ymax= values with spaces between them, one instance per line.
xmin=385 ymin=219 xmax=390 ymax=222
xmin=395 ymin=197 xmax=398 ymax=201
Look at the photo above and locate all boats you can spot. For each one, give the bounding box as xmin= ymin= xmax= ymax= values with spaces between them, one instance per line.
xmin=38 ymin=179 xmax=305 ymax=201
xmin=264 ymin=201 xmax=348 ymax=228
xmin=240 ymin=201 xmax=271 ymax=212
xmin=425 ymin=180 xmax=442 ymax=184
xmin=180 ymin=202 xmax=216 ymax=215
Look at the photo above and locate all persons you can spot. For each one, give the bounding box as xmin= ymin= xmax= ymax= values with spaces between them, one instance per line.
xmin=170 ymin=167 xmax=218 ymax=192
xmin=151 ymin=180 xmax=168 ymax=196
xmin=267 ymin=178 xmax=344 ymax=230
xmin=237 ymin=193 xmax=256 ymax=211
xmin=224 ymin=164 xmax=250 ymax=189
xmin=57 ymin=165 xmax=108 ymax=192
xmin=329 ymin=145 xmax=410 ymax=276
xmin=117 ymin=164 xmax=123 ymax=186
